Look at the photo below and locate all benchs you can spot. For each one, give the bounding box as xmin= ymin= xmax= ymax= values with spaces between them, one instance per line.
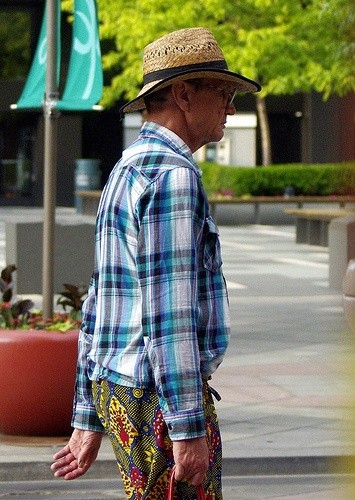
xmin=286 ymin=208 xmax=355 ymax=249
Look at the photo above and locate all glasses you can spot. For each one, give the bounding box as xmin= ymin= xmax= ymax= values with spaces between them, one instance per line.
xmin=201 ymin=87 xmax=237 ymax=104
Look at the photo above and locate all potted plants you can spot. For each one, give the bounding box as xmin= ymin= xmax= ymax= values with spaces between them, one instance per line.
xmin=0 ymin=265 xmax=84 ymax=437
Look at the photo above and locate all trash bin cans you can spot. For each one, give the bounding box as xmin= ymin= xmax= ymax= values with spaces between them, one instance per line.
xmin=73 ymin=159 xmax=102 ymax=215
xmin=16 ymin=158 xmax=31 ymax=193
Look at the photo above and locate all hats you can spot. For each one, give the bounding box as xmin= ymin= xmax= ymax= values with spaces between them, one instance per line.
xmin=118 ymin=27 xmax=262 ymax=113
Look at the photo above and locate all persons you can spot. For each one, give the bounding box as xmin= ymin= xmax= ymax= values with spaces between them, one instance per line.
xmin=48 ymin=26 xmax=262 ymax=500
